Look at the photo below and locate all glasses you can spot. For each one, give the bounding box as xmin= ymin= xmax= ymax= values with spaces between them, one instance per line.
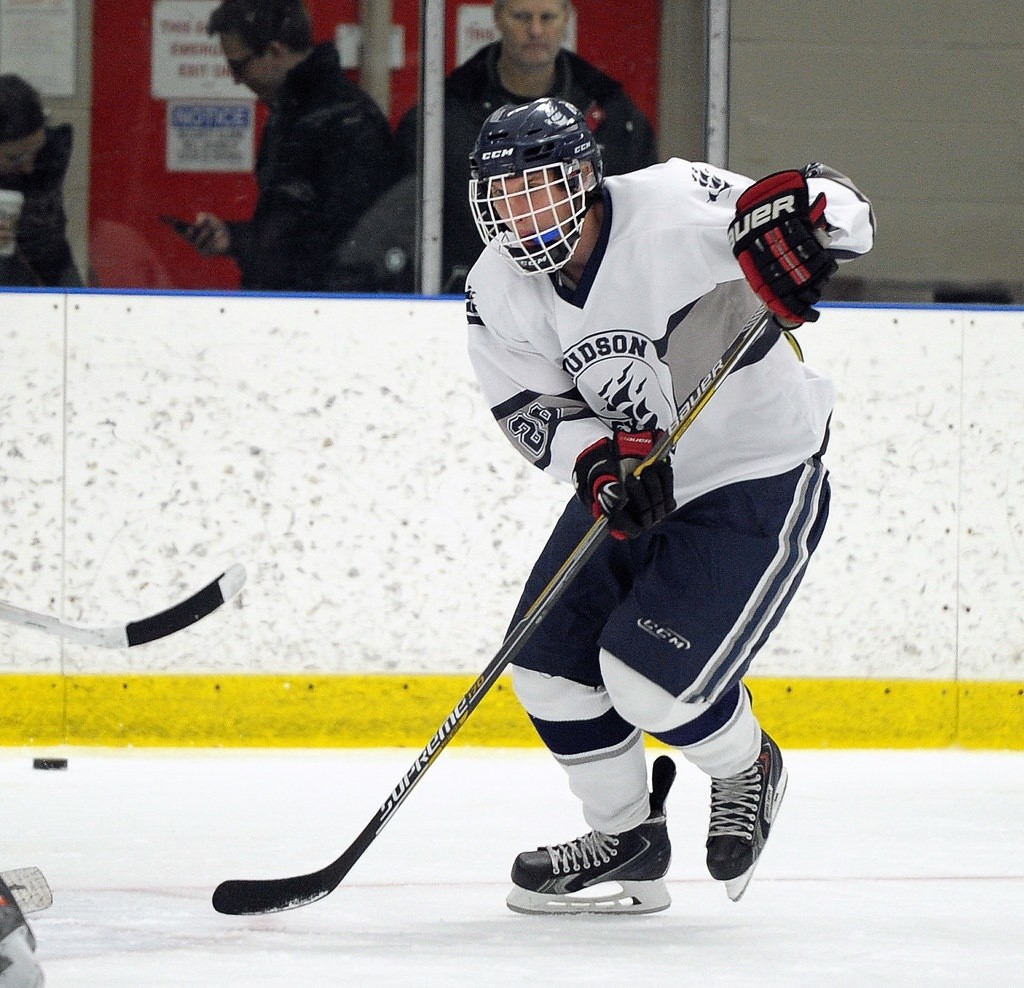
xmin=226 ymin=44 xmax=270 ymax=83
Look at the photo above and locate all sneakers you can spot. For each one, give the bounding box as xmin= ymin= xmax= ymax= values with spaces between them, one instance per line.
xmin=707 ymin=686 xmax=790 ymax=903
xmin=506 ymin=755 xmax=677 ymax=916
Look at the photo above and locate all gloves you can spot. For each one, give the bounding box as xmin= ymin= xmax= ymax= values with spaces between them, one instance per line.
xmin=727 ymin=170 xmax=839 ymax=322
xmin=566 ymin=428 xmax=674 ymax=543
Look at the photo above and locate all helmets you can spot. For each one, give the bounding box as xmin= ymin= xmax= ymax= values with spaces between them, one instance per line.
xmin=468 ymin=98 xmax=603 ymax=274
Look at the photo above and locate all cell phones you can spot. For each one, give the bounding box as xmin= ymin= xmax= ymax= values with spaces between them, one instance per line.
xmin=159 ymin=214 xmax=202 ymax=235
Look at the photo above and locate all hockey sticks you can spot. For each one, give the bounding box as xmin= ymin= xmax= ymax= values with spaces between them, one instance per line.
xmin=0 ymin=561 xmax=249 ymax=650
xmin=212 ymin=227 xmax=833 ymax=917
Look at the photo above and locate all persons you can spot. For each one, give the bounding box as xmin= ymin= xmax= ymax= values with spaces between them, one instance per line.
xmin=465 ymin=96 xmax=875 ymax=915
xmin=1 ymin=72 xmax=82 ymax=288
xmin=182 ymin=1 xmax=661 ymax=296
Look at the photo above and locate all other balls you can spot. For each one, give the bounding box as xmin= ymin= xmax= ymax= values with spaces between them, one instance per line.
xmin=32 ymin=757 xmax=68 ymax=771
